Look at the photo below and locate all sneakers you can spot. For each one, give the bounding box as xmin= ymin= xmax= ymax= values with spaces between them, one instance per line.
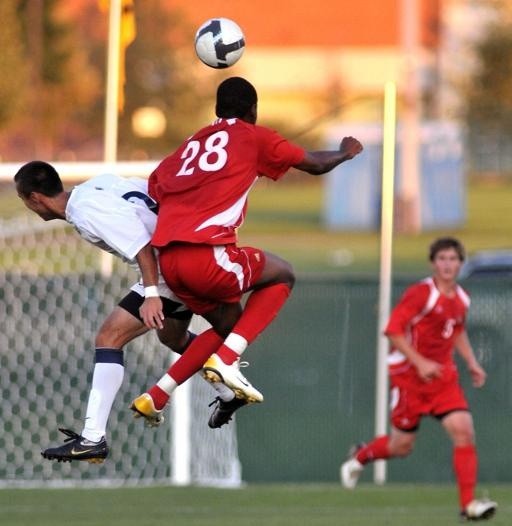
xmin=41 ymin=428 xmax=109 ymax=464
xmin=204 ymin=353 xmax=264 ymax=403
xmin=128 ymin=391 xmax=167 ymax=427
xmin=208 ymin=394 xmax=247 ymax=428
xmin=340 ymin=441 xmax=366 ymax=492
xmin=460 ymin=499 xmax=497 ymax=520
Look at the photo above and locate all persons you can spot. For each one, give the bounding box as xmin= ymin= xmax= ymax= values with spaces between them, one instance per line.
xmin=131 ymin=76 xmax=361 ymax=427
xmin=339 ymin=238 xmax=499 ymax=517
xmin=13 ymin=161 xmax=249 ymax=463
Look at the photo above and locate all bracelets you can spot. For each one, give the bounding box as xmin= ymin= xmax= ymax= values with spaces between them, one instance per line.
xmin=143 ymin=285 xmax=160 ymax=297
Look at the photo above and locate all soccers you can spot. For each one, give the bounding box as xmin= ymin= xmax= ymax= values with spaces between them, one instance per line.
xmin=194 ymin=18 xmax=245 ymax=68
